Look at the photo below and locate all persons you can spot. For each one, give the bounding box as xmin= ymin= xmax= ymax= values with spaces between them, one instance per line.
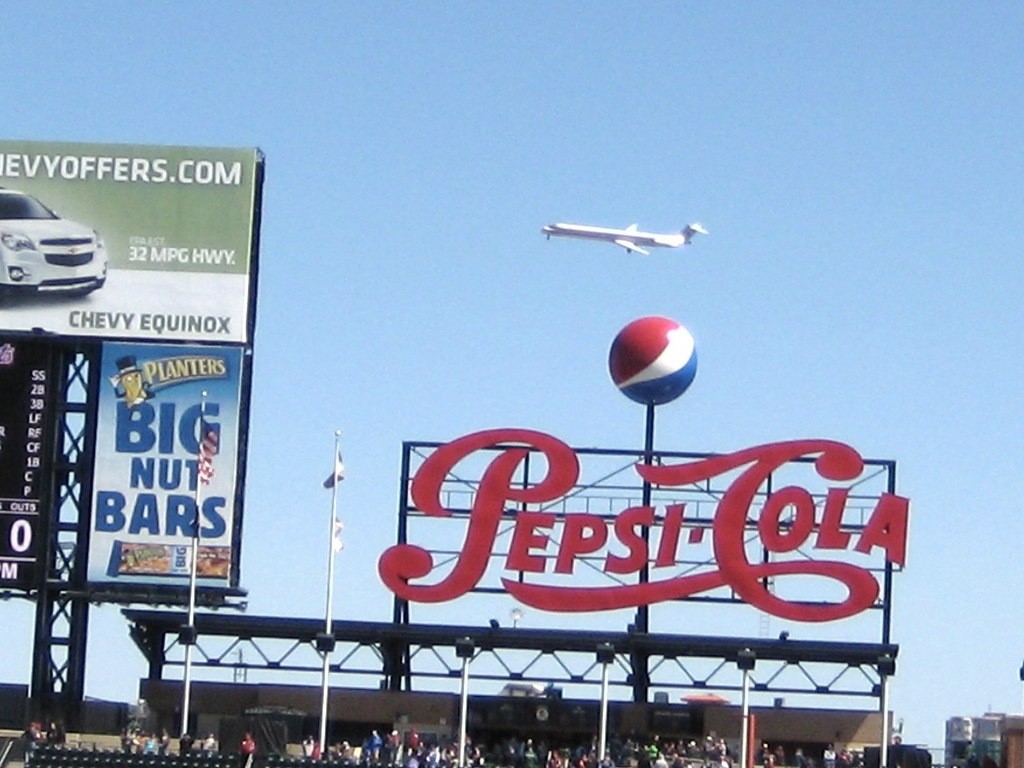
xmin=759 ymin=742 xmax=852 ymax=768
xmin=340 ymin=729 xmax=726 ymax=768
xmin=19 ymin=720 xmax=66 ymax=750
xmin=239 ymin=731 xmax=255 ymax=768
xmin=204 ymin=732 xmax=218 ymax=751
xmin=119 ymin=727 xmax=172 ymax=753
xmin=301 ymin=734 xmax=320 ymax=759
xmin=179 ymin=733 xmax=194 ymax=757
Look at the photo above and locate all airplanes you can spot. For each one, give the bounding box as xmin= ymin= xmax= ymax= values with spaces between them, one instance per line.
xmin=539 ymin=215 xmax=705 ymax=256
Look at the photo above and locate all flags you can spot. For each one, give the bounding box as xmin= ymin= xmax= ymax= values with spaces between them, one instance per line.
xmin=323 ymin=451 xmax=345 ymax=488
xmin=196 ymin=420 xmax=218 ymax=488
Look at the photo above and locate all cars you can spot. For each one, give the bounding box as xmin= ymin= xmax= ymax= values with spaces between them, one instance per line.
xmin=0 ymin=186 xmax=109 ymax=301
